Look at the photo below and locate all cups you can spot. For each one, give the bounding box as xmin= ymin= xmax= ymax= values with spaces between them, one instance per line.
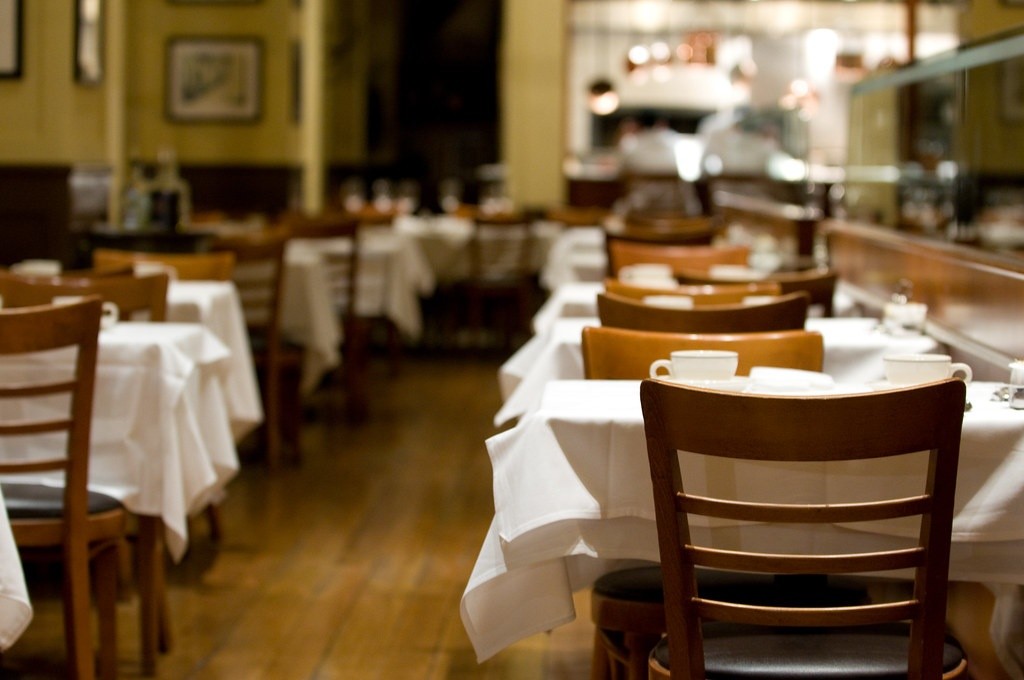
xmin=648 ymin=349 xmax=738 ymax=383
xmin=882 ymin=354 xmax=973 ymax=390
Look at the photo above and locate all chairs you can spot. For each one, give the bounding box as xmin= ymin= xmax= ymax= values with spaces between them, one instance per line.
xmin=0 ymin=203 xmax=400 ymax=673
xmin=582 ymin=213 xmax=967 ymax=680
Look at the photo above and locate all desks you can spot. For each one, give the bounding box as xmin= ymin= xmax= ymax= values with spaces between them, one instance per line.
xmin=0 ymin=216 xmax=476 ymax=680
xmin=461 ymin=225 xmax=1024 ymax=680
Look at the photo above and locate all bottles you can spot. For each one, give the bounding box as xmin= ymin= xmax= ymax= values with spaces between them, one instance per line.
xmin=1008 ymin=361 xmax=1024 ymax=410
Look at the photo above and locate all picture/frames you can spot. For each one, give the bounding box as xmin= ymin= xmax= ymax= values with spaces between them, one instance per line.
xmin=74 ymin=0 xmax=103 ymax=83
xmin=0 ymin=0 xmax=24 ymax=79
xmin=161 ymin=33 xmax=265 ymax=125
